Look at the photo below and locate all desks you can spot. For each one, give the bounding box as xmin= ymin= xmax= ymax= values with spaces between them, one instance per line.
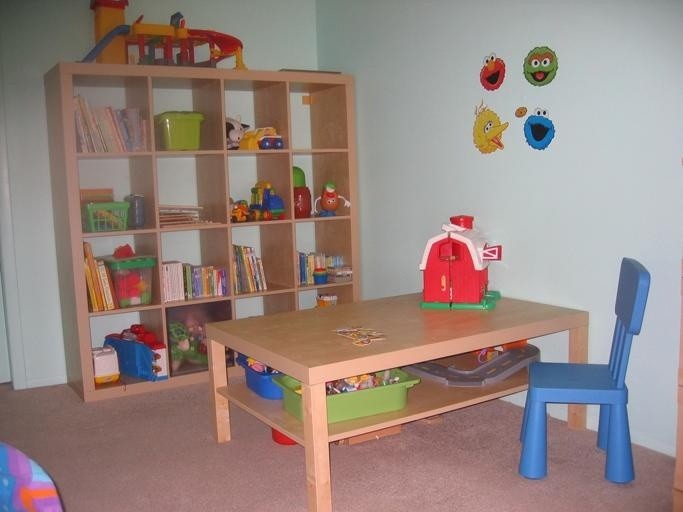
xmin=204 ymin=292 xmax=589 ymax=511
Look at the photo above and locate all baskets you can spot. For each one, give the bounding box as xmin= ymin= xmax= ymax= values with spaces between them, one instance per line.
xmin=82 ymin=201 xmax=130 ymax=233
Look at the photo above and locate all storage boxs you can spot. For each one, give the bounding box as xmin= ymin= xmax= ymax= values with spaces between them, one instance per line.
xmin=235 ymin=355 xmax=420 ymax=423
xmin=98 ymin=254 xmax=155 ymax=307
xmin=154 ymin=111 xmax=204 ymax=151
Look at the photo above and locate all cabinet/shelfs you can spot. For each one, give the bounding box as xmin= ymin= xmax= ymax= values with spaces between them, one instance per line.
xmin=40 ymin=62 xmax=362 ymax=402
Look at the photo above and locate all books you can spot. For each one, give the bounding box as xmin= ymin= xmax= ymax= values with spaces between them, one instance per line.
xmin=297 ymin=250 xmax=352 ymax=285
xmin=73 ymin=92 xmax=150 ymax=154
xmin=162 ymin=261 xmax=227 ymax=302
xmin=83 ymin=241 xmax=119 ymax=312
xmin=231 ymin=244 xmax=268 ymax=295
xmin=159 ymin=204 xmax=204 ymax=228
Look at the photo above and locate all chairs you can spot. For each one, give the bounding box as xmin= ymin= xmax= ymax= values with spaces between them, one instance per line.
xmin=517 ymin=256 xmax=651 ymax=485
